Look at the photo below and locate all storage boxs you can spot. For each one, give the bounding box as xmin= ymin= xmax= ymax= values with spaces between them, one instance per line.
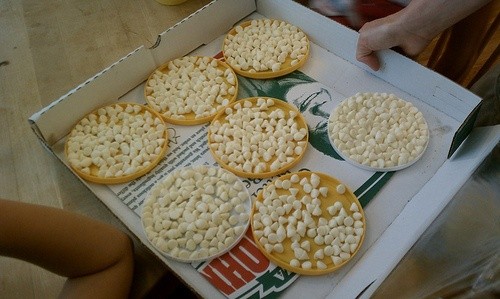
xmin=27 ymin=0 xmax=500 ymax=299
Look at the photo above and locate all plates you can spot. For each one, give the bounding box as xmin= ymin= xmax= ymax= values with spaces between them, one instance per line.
xmin=250 ymin=172 xmax=368 ymax=275
xmin=142 ymin=53 xmax=238 ymax=125
xmin=207 ymin=95 xmax=309 ymax=178
xmin=141 ymin=165 xmax=253 ymax=262
xmin=327 ymin=89 xmax=430 ymax=172
xmin=64 ymin=101 xmax=169 ymax=185
xmin=221 ymin=17 xmax=311 ymax=79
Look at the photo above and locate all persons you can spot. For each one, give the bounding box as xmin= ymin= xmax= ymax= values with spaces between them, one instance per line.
xmin=356 ymin=0 xmax=500 ymax=127
xmin=0 ymin=198 xmax=135 ymax=299
